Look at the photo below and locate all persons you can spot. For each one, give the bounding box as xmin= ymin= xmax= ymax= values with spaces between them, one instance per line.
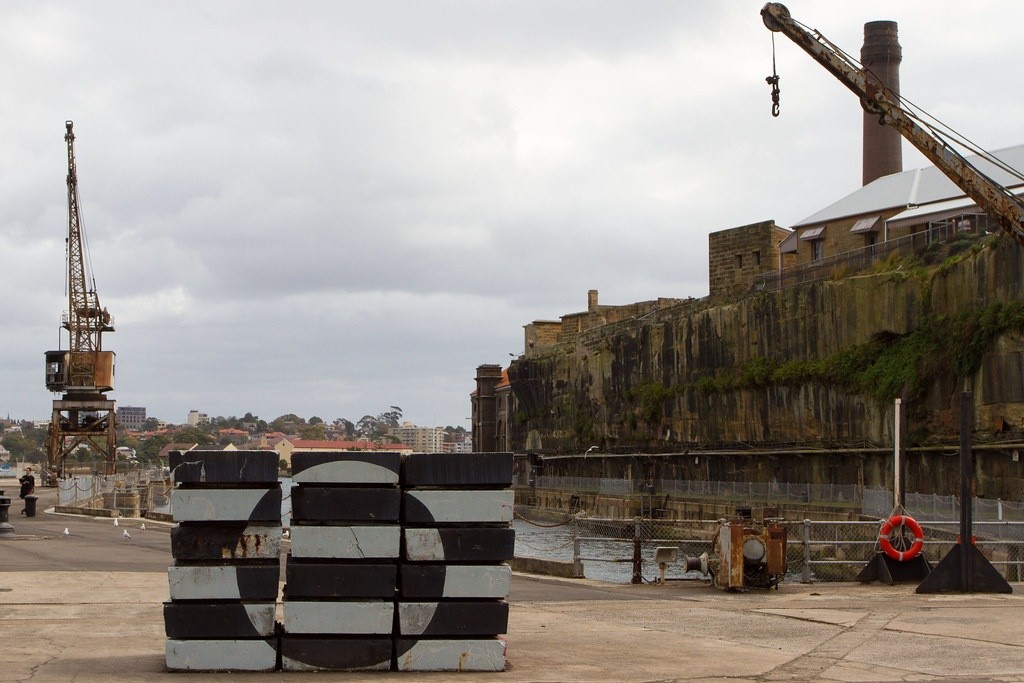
xmin=19 ymin=467 xmax=34 ymax=498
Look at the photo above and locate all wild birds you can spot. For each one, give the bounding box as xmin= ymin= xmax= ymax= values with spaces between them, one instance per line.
xmin=63 ymin=528 xmax=70 ymax=535
xmin=140 ymin=522 xmax=145 ymax=532
xmin=113 ymin=518 xmax=118 ymax=527
xmin=123 ymin=529 xmax=131 ymax=539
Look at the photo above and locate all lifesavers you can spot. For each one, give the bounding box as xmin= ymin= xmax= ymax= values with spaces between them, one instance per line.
xmin=879 ymin=515 xmax=924 ymax=562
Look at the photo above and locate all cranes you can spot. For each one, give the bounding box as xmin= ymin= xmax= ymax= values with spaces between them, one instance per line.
xmin=42 ymin=121 xmax=119 ymax=488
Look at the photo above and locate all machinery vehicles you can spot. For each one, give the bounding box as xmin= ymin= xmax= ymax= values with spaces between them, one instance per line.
xmin=761 ymin=2 xmax=1023 ymax=244
